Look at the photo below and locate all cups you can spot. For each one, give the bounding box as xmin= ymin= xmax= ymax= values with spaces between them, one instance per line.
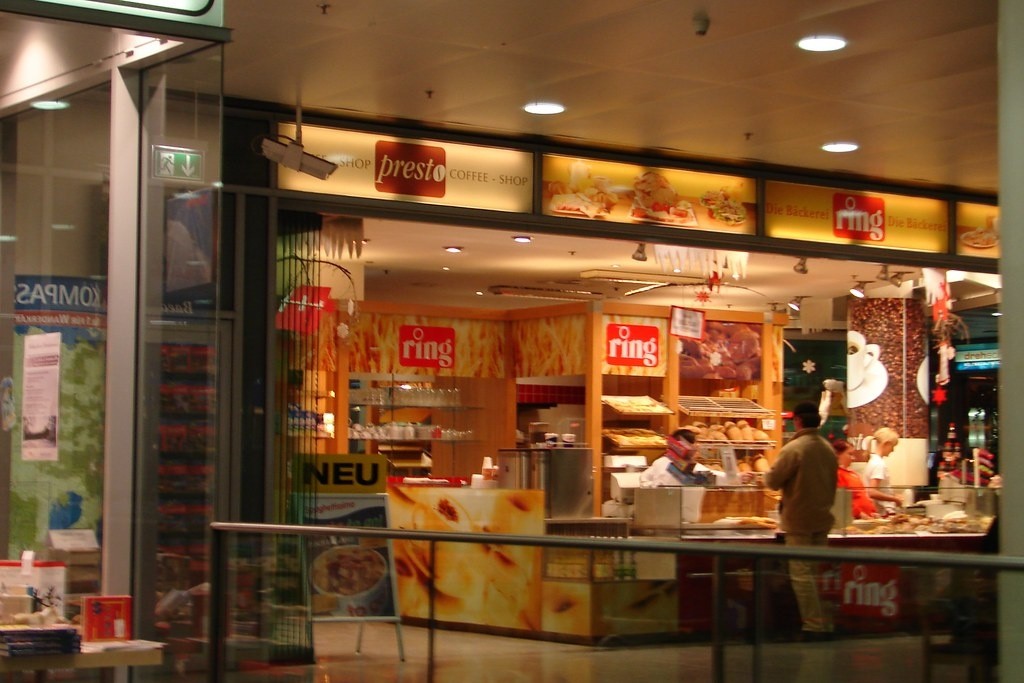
xmin=562 ymin=434 xmax=575 ymax=447
xmin=349 ymin=388 xmax=475 ymax=439
xmin=545 ymin=434 xmax=558 ymax=447
xmin=847 ymin=330 xmax=880 ymax=390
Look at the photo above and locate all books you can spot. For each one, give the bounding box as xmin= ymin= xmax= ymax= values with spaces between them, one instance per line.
xmin=0 ymin=624 xmax=82 ymax=657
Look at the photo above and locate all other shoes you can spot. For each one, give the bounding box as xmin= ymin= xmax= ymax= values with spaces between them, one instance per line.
xmin=791 ymin=629 xmax=836 ymax=643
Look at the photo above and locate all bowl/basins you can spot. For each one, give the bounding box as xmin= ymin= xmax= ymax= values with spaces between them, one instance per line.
xmin=311 ymin=545 xmax=387 ymax=599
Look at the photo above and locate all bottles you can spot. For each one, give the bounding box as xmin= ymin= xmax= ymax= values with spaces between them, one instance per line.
xmin=546 ymin=535 xmax=633 ymax=581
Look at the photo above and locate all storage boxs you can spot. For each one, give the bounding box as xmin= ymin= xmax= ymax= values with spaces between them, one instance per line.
xmin=81 ymin=595 xmax=131 ymax=641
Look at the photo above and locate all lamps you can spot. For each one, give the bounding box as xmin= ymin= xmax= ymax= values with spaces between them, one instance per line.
xmin=795 ymin=256 xmax=808 ymax=275
xmin=889 ymin=272 xmax=903 ymax=287
xmin=878 ymin=267 xmax=888 ymax=280
xmin=850 ymin=280 xmax=865 ymax=299
xmin=631 ymin=244 xmax=647 ymax=263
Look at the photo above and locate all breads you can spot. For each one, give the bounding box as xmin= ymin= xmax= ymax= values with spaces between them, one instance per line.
xmin=702 ymin=453 xmax=772 ymax=473
xmin=678 ymin=322 xmax=760 ymax=379
xmin=682 ymin=419 xmax=771 ymax=441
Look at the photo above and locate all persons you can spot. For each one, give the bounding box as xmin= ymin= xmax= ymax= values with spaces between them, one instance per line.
xmin=639 ymin=429 xmax=756 ymax=489
xmin=831 ymin=439 xmax=879 ymax=519
xmin=861 ymin=427 xmax=903 ymax=515
xmin=765 ymin=402 xmax=839 ymax=640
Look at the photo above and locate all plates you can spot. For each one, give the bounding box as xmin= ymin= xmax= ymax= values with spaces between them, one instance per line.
xmin=846 ymin=354 xmax=888 ymax=408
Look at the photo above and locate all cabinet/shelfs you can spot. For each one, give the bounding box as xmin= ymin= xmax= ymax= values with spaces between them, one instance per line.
xmin=321 ymin=296 xmax=998 ymax=642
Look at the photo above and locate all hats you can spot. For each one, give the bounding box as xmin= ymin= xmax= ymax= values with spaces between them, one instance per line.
xmin=793 ymin=402 xmax=820 ymax=415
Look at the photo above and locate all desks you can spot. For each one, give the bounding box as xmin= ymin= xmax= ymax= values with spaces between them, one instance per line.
xmin=0 ymin=637 xmax=164 ymax=683
